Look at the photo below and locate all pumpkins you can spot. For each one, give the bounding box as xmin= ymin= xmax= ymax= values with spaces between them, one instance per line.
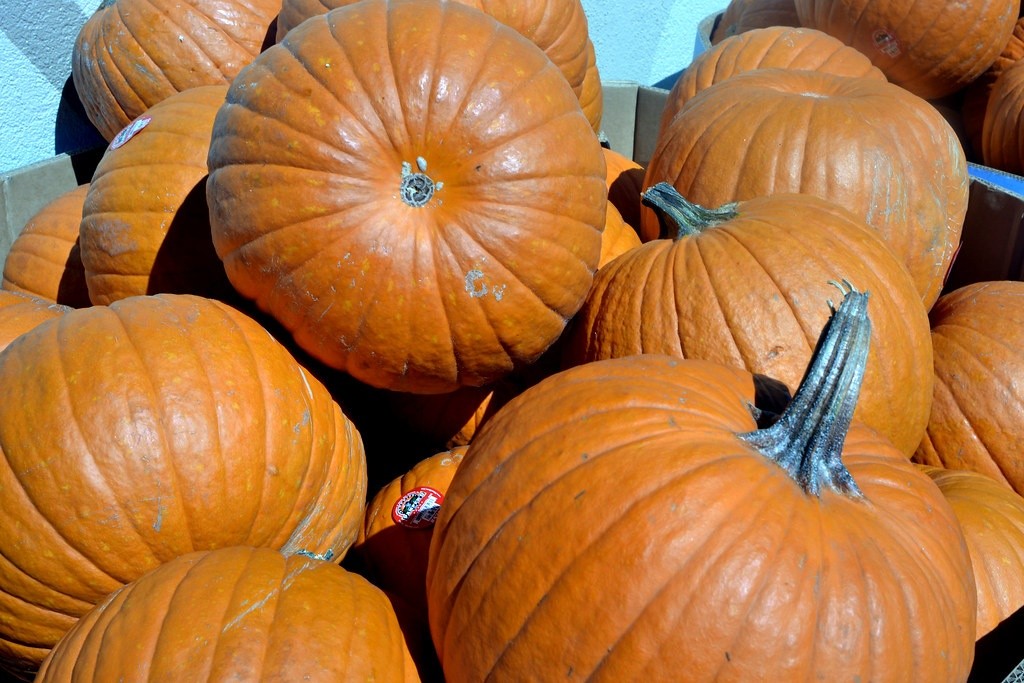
xmin=0 ymin=0 xmax=1024 ymax=681
